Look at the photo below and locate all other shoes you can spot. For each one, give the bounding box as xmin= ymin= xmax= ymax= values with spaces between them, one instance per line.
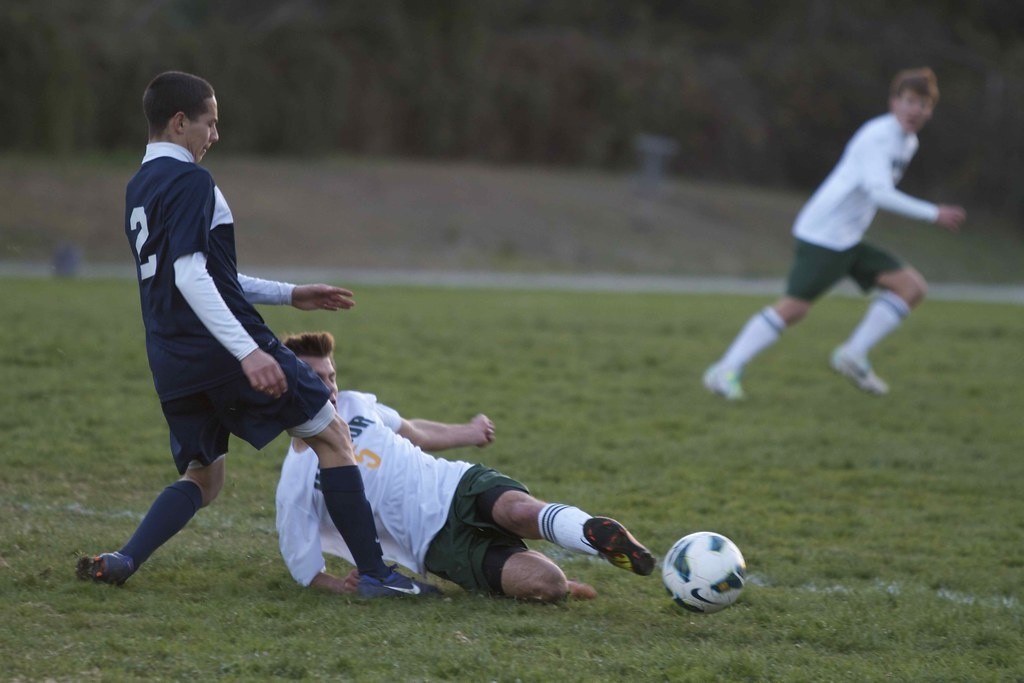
xmin=829 ymin=345 xmax=890 ymax=399
xmin=704 ymin=366 xmax=747 ymax=402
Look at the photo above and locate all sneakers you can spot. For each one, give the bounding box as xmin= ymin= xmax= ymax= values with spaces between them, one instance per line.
xmin=357 ymin=564 xmax=443 ymax=599
xmin=75 ymin=550 xmax=135 ymax=585
xmin=581 ymin=516 xmax=657 ymax=576
xmin=568 ymin=581 xmax=598 ymax=600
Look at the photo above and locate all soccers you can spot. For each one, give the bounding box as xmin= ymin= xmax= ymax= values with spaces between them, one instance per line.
xmin=661 ymin=531 xmax=747 ymax=614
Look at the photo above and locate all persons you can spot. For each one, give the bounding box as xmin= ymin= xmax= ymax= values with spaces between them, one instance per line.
xmin=76 ymin=72 xmax=442 ymax=599
xmin=702 ymin=68 xmax=969 ymax=401
xmin=273 ymin=333 xmax=657 ymax=603
